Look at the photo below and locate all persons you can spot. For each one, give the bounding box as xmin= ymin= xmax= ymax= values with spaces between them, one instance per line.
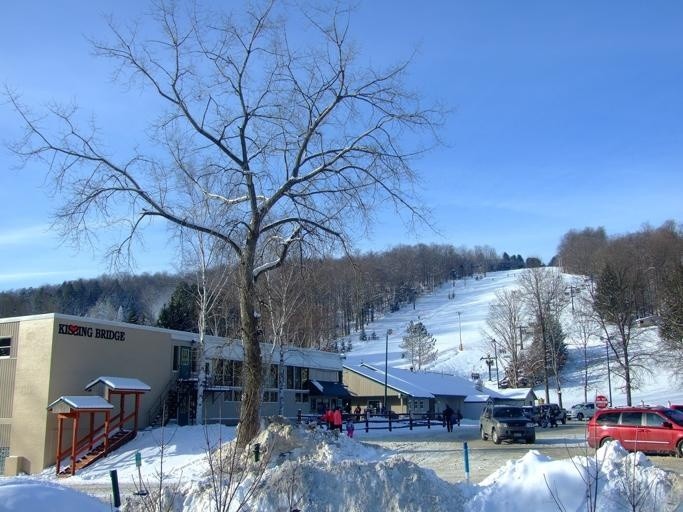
xmin=333 ymin=405 xmax=342 ymax=434
xmin=319 ymin=407 xmax=333 ymax=430
xmin=547 ymin=406 xmax=558 ymax=427
xmin=353 ymin=404 xmax=360 ymax=421
xmin=343 ymin=402 xmax=350 ymax=421
xmin=442 ymin=403 xmax=454 ymax=432
xmin=345 ymin=416 xmax=354 ymax=438
xmin=537 ymin=405 xmax=547 ymax=428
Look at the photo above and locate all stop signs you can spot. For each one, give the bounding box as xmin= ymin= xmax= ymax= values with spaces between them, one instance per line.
xmin=595 ymin=395 xmax=608 ymax=408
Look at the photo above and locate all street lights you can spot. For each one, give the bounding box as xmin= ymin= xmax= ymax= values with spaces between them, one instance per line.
xmin=491 ymin=339 xmax=498 ymax=381
xmin=382 ymin=329 xmax=392 ymax=415
xmin=600 ymin=335 xmax=615 ymax=406
xmin=456 ymin=311 xmax=463 ymax=351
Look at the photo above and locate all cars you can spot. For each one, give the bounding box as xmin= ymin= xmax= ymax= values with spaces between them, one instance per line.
xmin=588 ymin=405 xmax=683 ymax=458
xmin=479 ymin=404 xmax=535 ymax=444
xmin=522 ymin=402 xmax=600 ymax=428
